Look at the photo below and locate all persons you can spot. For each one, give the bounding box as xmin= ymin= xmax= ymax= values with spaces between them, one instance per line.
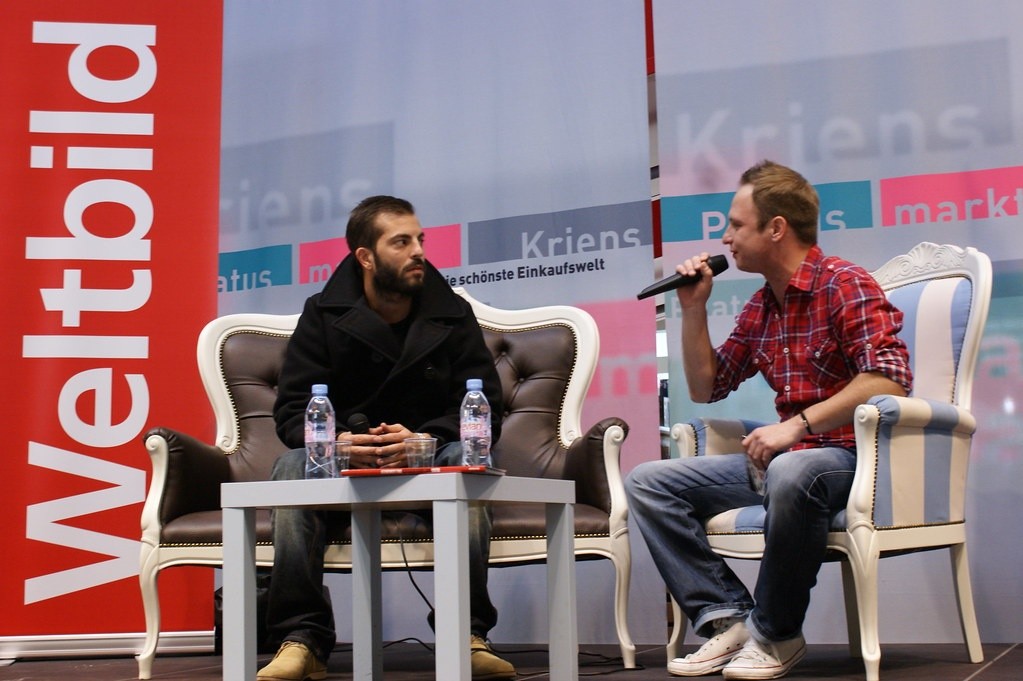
xmin=622 ymin=156 xmax=913 ymax=678
xmin=254 ymin=194 xmax=518 ymax=681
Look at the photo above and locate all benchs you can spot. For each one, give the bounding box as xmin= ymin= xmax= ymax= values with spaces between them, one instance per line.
xmin=136 ymin=288 xmax=636 ymax=681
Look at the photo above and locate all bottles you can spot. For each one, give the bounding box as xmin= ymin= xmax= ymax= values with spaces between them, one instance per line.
xmin=303 ymin=384 xmax=336 ymax=479
xmin=459 ymin=379 xmax=492 ymax=467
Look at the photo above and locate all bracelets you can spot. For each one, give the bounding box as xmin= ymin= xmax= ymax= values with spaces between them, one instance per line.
xmin=799 ymin=411 xmax=813 ymax=435
xmin=416 ymin=432 xmax=426 ymax=458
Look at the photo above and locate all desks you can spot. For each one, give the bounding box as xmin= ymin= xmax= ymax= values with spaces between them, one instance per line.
xmin=219 ymin=472 xmax=579 ymax=681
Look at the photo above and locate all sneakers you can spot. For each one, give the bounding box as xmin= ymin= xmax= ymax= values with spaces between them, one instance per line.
xmin=722 ymin=628 xmax=808 ymax=680
xmin=471 ymin=633 xmax=516 ymax=680
xmin=256 ymin=640 xmax=327 ymax=681
xmin=667 ymin=614 xmax=752 ymax=676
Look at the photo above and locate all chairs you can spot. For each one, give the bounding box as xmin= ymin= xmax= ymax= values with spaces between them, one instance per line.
xmin=665 ymin=242 xmax=994 ymax=681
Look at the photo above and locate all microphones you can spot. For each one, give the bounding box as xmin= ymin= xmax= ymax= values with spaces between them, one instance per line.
xmin=347 ymin=412 xmax=381 ymax=469
xmin=636 ymin=254 xmax=730 ymax=301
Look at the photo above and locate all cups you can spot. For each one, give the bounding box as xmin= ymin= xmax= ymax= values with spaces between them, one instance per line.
xmin=404 ymin=437 xmax=438 ymax=467
xmin=334 ymin=441 xmax=352 ymax=478
